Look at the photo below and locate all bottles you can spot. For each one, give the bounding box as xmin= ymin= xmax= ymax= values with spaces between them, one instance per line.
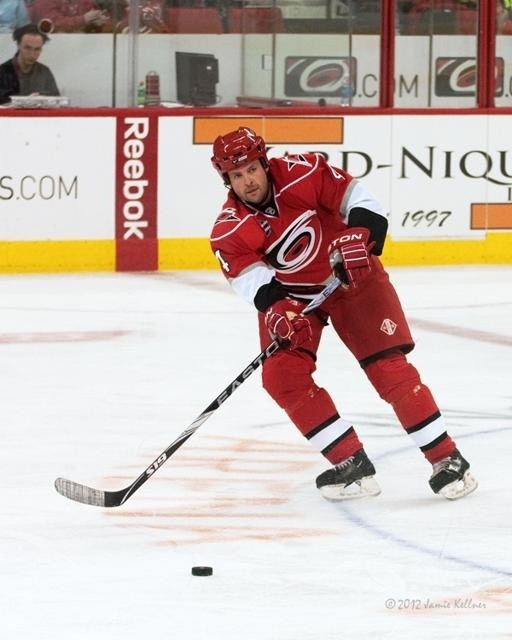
xmin=137 ymin=67 xmax=160 ymax=107
xmin=342 ymin=72 xmax=352 ymax=107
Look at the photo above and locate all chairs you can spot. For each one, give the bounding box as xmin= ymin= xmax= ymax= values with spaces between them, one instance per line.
xmin=164 ymin=7 xmax=223 ymax=34
xmin=403 ymin=0 xmax=477 ymax=35
xmin=227 ymin=8 xmax=286 ymax=33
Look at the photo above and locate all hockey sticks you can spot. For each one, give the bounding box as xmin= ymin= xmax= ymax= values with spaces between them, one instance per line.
xmin=54 ymin=276 xmax=340 ymax=507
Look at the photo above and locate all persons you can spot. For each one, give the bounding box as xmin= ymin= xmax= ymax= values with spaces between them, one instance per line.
xmin=0 ymin=24 xmax=62 ymax=108
xmin=0 ymin=1 xmax=172 ymax=35
xmin=206 ymin=125 xmax=469 ymax=491
xmin=474 ymin=1 xmax=511 ymax=35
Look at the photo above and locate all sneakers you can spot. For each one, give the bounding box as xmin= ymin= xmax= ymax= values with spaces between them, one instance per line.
xmin=428 ymin=447 xmax=470 ymax=492
xmin=316 ymin=447 xmax=376 ymax=487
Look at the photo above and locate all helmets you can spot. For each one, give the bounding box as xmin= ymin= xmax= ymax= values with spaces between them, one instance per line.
xmin=211 ymin=127 xmax=271 ymax=185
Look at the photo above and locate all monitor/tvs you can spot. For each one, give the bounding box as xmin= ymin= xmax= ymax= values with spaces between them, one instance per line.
xmin=176 ymin=52 xmax=219 ymax=106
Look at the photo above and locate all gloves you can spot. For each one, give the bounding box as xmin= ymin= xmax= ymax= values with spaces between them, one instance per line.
xmin=328 ymin=227 xmax=373 ymax=289
xmin=266 ymin=296 xmax=313 ymax=351
